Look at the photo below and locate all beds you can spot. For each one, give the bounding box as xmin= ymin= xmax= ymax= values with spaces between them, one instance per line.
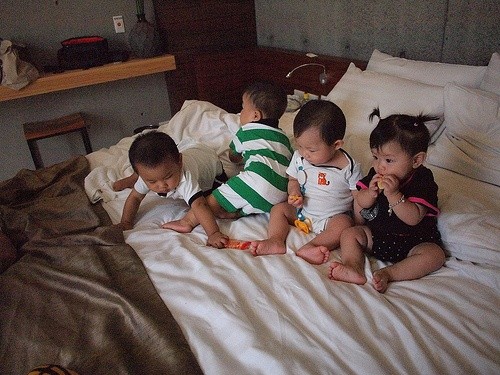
xmin=0 ymin=47 xmax=500 ymax=375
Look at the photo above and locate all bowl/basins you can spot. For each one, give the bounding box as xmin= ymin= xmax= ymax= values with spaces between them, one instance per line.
xmin=55 ymin=36 xmax=112 ymax=64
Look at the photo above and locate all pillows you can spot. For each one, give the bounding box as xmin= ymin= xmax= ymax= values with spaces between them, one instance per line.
xmin=424 ymin=81 xmax=500 ymax=187
xmin=479 ymin=51 xmax=500 ymax=97
xmin=365 ymin=48 xmax=488 ymax=90
xmin=324 ymin=61 xmax=447 ymax=146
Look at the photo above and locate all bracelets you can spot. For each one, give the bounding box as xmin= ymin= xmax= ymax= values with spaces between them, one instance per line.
xmin=388 ymin=194 xmax=404 ymax=215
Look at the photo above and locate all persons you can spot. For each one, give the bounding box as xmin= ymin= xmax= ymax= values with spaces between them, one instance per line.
xmin=111 ymin=130 xmax=229 ymax=249
xmin=327 ymin=106 xmax=445 ymax=294
xmin=250 ymin=99 xmax=366 ymax=264
xmin=161 ymin=81 xmax=294 ymax=233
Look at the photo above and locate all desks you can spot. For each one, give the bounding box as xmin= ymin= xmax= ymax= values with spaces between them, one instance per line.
xmin=0 ymin=52 xmax=177 ymax=101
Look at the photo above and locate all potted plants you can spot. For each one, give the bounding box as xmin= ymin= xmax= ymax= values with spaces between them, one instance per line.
xmin=128 ymin=0 xmax=162 ymax=58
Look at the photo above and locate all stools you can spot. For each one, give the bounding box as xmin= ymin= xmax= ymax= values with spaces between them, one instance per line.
xmin=22 ymin=113 xmax=93 ymax=170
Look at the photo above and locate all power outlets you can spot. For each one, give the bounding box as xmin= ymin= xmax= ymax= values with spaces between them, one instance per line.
xmin=112 ymin=16 xmax=125 ymax=33
